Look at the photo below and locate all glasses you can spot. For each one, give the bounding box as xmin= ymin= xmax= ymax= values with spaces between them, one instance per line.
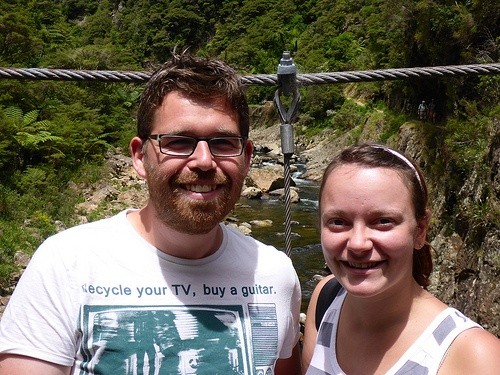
xmin=147 ymin=133 xmax=248 ymax=157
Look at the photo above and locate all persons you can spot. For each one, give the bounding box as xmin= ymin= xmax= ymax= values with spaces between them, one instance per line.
xmin=301 ymin=144 xmax=500 ymax=374
xmin=1 ymin=53 xmax=303 ymax=374
